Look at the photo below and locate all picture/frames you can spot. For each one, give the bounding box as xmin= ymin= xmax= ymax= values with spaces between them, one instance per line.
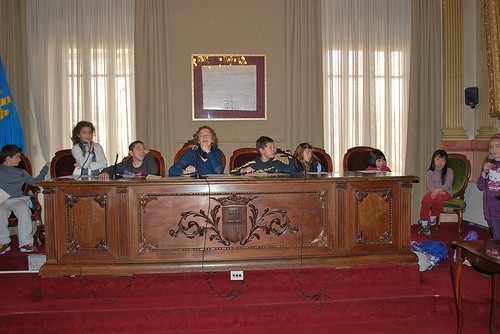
xmin=191 ymin=53 xmax=267 ymax=122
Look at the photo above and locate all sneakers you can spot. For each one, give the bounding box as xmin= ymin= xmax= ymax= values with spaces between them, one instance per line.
xmin=418 ymin=217 xmax=437 ymax=226
xmin=21 ymin=245 xmax=37 ymax=252
xmin=422 ymin=225 xmax=431 ymax=236
xmin=0 ymin=243 xmax=11 ymax=255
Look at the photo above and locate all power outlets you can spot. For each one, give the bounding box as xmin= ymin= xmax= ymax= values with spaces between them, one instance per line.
xmin=229 ymin=271 xmax=244 ymax=280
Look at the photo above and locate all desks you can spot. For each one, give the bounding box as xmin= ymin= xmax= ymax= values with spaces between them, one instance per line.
xmin=36 ymin=171 xmax=418 ymax=277
xmin=449 ymin=238 xmax=500 ymax=334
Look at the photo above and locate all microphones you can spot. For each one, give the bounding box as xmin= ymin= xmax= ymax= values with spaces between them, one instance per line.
xmin=112 ymin=153 xmax=118 ymax=179
xmin=276 ymin=148 xmax=306 ymax=178
xmin=485 ymin=153 xmax=495 ymax=173
xmin=81 ymin=150 xmax=90 ymax=180
xmin=190 ymin=144 xmax=207 ymax=179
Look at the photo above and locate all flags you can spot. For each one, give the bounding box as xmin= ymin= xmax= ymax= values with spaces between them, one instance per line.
xmin=0 ymin=58 xmax=26 ymax=157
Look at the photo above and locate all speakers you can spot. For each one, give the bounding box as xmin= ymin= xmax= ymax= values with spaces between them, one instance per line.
xmin=465 ymin=87 xmax=478 ymax=105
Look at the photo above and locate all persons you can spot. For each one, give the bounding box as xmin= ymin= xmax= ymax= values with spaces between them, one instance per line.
xmin=230 ymin=135 xmax=295 ymax=174
xmin=420 ymin=149 xmax=454 ymax=235
xmin=97 ymin=141 xmax=158 ymax=179
xmin=476 ymin=134 xmax=500 ymax=241
xmin=366 ymin=149 xmax=390 ymax=171
xmin=293 ymin=143 xmax=326 ymax=172
xmin=169 ymin=126 xmax=223 ymax=176
xmin=0 ymin=144 xmax=51 ymax=256
xmin=71 ymin=120 xmax=107 ymax=175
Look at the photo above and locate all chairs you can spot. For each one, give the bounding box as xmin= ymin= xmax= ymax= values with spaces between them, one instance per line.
xmin=441 ymin=153 xmax=471 ymax=233
xmin=311 ymin=148 xmax=333 ymax=172
xmin=49 ymin=150 xmax=76 ymax=181
xmin=174 ymin=142 xmax=227 ymax=172
xmin=122 ymin=149 xmax=165 ymax=176
xmin=229 ymin=148 xmax=260 ymax=173
xmin=342 ymin=146 xmax=380 ymax=171
xmin=8 ymin=151 xmax=43 ymax=246
